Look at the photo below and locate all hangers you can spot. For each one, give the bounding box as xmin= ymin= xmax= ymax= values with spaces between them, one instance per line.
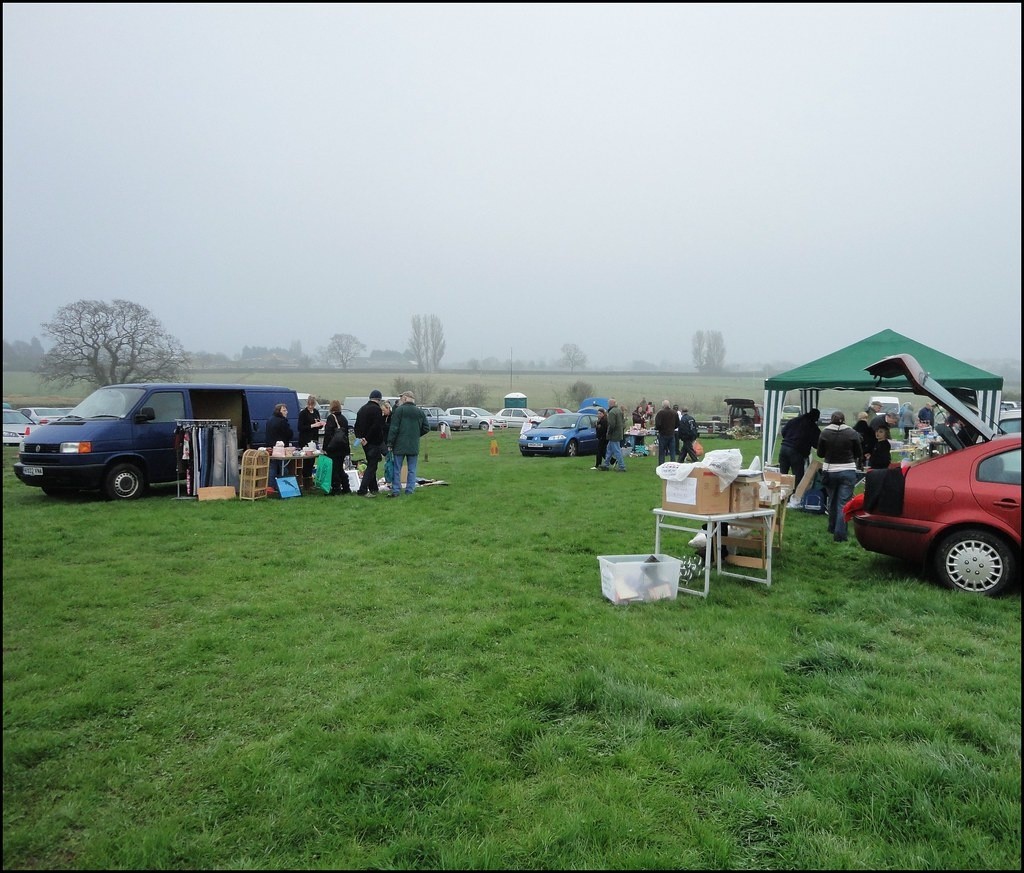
xmin=173 ymin=420 xmax=233 ymax=431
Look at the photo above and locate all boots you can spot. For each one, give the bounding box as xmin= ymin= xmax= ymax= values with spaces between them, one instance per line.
xmin=302 ymin=477 xmax=320 ymax=494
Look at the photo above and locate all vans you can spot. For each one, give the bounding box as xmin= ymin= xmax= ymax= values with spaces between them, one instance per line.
xmin=297 ymin=393 xmax=321 ymax=410
xmin=343 ymin=397 xmax=402 ymax=416
xmin=724 ymin=399 xmax=764 ymax=431
xmin=868 ymin=396 xmax=900 ymax=416
xmin=14 ymin=383 xmax=301 ymax=501
xmin=1000 ymin=401 xmax=1020 ymax=409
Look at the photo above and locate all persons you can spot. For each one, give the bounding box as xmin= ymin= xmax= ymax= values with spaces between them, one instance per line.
xmin=264 ymin=390 xmax=352 ymax=496
xmin=590 ymin=397 xmax=700 ymax=472
xmin=777 ymin=401 xmax=934 ymax=542
xmin=354 ymin=389 xmax=431 ymax=498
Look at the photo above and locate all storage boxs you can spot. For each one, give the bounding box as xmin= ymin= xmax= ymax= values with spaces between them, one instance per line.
xmin=660 ymin=463 xmax=731 ymax=515
xmin=731 ymin=480 xmax=759 ymax=514
xmin=759 ymin=468 xmax=796 ymax=507
xmin=597 ymin=554 xmax=684 ymax=606
xmin=889 ymin=440 xmax=903 ymax=449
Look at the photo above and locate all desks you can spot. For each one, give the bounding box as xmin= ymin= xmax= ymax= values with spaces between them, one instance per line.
xmin=624 ymin=433 xmax=648 ymax=454
xmin=652 ymin=506 xmax=776 ymax=597
xmin=269 ymin=454 xmax=318 ymax=477
xmin=890 ymin=449 xmax=915 ymax=463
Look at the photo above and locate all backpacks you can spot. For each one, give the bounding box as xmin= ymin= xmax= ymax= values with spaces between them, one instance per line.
xmin=326 ymin=413 xmax=347 ymax=453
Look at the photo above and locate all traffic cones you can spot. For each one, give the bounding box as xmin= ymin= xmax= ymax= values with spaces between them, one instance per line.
xmin=489 ymin=438 xmax=500 ymax=455
xmin=488 ymin=420 xmax=493 ymax=436
xmin=439 ymin=424 xmax=446 ymax=439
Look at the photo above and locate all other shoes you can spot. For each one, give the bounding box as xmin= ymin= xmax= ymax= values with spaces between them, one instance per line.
xmin=364 ymin=491 xmax=382 ymax=498
xmin=590 ymin=467 xmax=597 ymax=469
xmin=386 ymin=493 xmax=397 ymax=497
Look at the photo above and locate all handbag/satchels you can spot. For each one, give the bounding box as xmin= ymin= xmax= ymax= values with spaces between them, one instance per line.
xmin=383 ymin=450 xmax=393 ymax=483
xmin=314 ymin=455 xmax=333 ymax=493
xmin=817 ymin=467 xmax=828 ymax=485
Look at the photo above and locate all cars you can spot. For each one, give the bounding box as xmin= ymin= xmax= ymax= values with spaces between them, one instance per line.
xmin=518 ymin=397 xmax=610 ymax=458
xmin=2 ymin=403 xmax=38 ymax=446
xmin=419 ymin=407 xmax=472 ymax=432
xmin=854 ymin=354 xmax=1022 ymax=599
xmin=445 ymin=407 xmax=509 ymax=431
xmin=781 ymin=405 xmax=801 ymax=423
xmin=495 ymin=407 xmax=546 ymax=429
xmin=316 ymin=404 xmax=357 ymax=434
xmin=16 ymin=407 xmax=75 ymax=426
xmin=995 ymin=409 xmax=1022 ymax=433
xmin=818 ymin=408 xmax=841 ymax=424
xmin=535 ymin=408 xmax=573 ymax=417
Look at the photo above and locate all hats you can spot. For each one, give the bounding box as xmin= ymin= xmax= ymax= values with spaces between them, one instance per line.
xmin=369 ymin=389 xmax=382 ymax=398
xmin=399 ymin=391 xmax=415 ymax=398
xmin=871 ymin=400 xmax=883 ymax=407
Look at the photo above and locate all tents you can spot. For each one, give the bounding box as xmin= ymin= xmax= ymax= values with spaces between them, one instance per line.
xmin=761 ymin=328 xmax=1004 ymax=483
xmin=799 ymin=385 xmax=981 ymax=468
